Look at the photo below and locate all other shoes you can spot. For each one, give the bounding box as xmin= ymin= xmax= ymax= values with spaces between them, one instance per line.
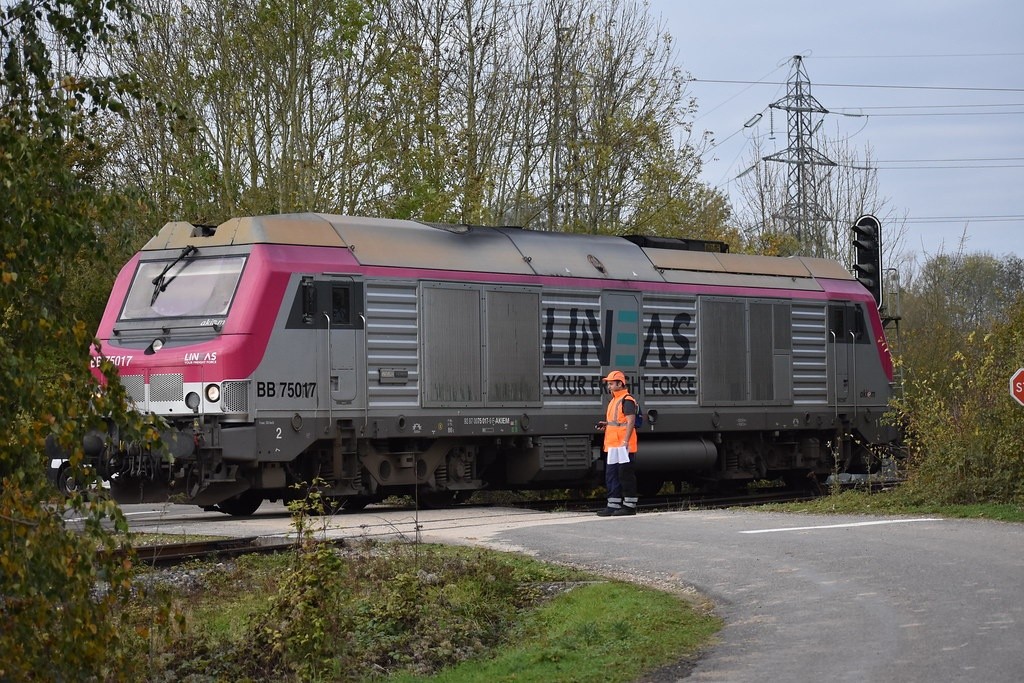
xmin=611 ymin=509 xmax=636 ymax=516
xmin=597 ymin=506 xmax=623 ymax=516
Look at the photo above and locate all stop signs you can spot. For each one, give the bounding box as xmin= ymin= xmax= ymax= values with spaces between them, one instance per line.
xmin=1009 ymin=368 xmax=1024 ymax=406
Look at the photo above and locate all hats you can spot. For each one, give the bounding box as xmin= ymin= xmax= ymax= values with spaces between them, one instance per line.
xmin=603 ymin=371 xmax=626 ymax=385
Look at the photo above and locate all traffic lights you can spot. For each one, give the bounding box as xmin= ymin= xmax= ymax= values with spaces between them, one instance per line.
xmin=850 ymin=213 xmax=883 ymax=312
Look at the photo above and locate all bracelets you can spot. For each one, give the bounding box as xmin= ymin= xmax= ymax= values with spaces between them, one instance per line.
xmin=622 ymin=440 xmax=629 ymax=444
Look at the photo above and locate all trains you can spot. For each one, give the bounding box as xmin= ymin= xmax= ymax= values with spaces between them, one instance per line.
xmin=45 ymin=211 xmax=907 ymax=517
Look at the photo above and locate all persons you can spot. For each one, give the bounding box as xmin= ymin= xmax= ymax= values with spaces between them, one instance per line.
xmin=595 ymin=370 xmax=640 ymax=516
